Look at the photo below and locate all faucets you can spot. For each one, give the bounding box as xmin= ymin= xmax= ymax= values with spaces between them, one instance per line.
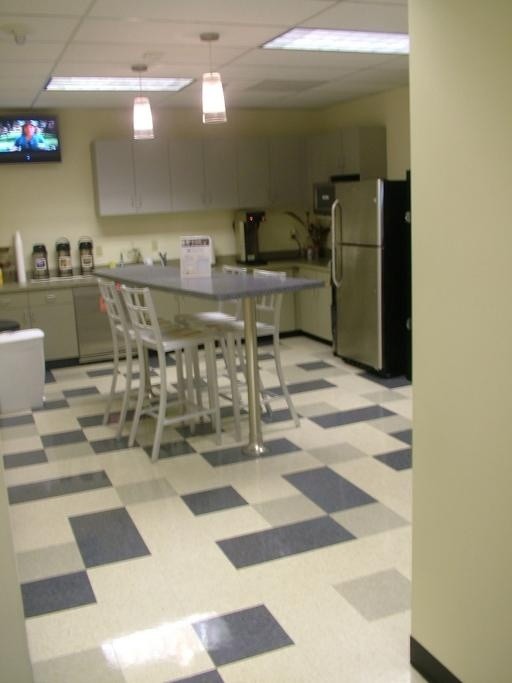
xmin=290 ymin=233 xmax=303 ymax=253
xmin=157 ymin=251 xmax=168 ymax=266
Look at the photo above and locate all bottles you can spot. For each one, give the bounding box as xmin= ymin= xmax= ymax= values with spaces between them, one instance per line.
xmin=78 ymin=235 xmax=95 ymax=275
xmin=54 ymin=237 xmax=74 ymax=277
xmin=31 ymin=243 xmax=50 ymax=280
xmin=0 ymin=247 xmax=16 ymax=283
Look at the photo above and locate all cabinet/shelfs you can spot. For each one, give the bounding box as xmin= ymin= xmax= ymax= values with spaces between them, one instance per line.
xmin=91 ymin=126 xmax=387 ymax=218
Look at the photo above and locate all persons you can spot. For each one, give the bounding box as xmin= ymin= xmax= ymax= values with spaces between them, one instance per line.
xmin=14 ymin=120 xmax=43 ymax=152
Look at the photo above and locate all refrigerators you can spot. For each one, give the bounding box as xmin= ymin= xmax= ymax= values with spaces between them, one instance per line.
xmin=331 ymin=178 xmax=412 ymax=378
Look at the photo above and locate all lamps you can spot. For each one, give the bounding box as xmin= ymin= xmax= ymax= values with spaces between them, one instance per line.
xmin=198 ymin=34 xmax=228 ymax=127
xmin=126 ymin=66 xmax=159 ymax=143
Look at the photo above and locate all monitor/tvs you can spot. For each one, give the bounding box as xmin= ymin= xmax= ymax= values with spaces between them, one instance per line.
xmin=0 ymin=113 xmax=62 ymax=163
